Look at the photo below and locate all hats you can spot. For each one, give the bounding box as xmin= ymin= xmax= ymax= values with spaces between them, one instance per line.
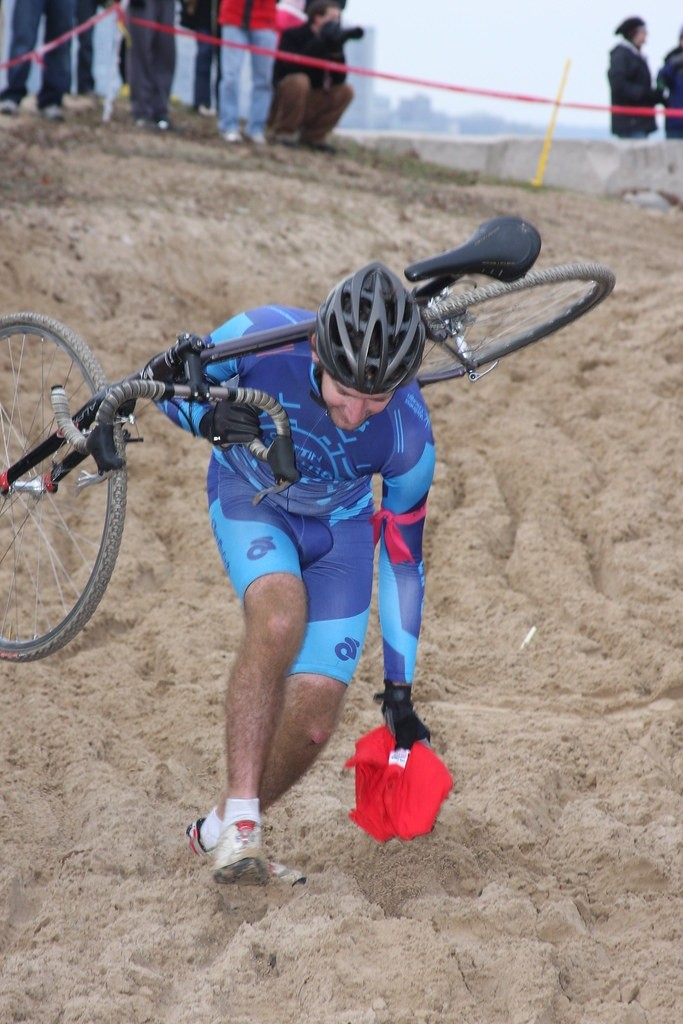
xmin=614 ymin=18 xmax=646 ymax=35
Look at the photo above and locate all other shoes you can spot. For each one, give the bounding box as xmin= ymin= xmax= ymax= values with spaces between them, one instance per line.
xmin=0 ymin=99 xmax=17 ymax=115
xmin=307 ymin=141 xmax=334 ymax=152
xmin=253 ymin=133 xmax=267 ymax=145
xmin=227 ymin=129 xmax=242 ymax=141
xmin=45 ymin=105 xmax=64 ymax=121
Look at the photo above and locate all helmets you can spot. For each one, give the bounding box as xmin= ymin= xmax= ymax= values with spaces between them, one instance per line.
xmin=313 ymin=261 xmax=427 ymax=392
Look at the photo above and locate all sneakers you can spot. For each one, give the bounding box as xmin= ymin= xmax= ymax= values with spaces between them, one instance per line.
xmin=186 ymin=818 xmax=306 ymax=887
xmin=213 ymin=819 xmax=267 ymax=886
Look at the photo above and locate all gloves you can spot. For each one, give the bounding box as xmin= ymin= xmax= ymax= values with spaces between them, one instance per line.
xmin=200 ymin=401 xmax=262 ymax=451
xmin=322 ymin=22 xmax=343 ymax=53
xmin=646 ymin=89 xmax=663 ymax=108
xmin=374 ymin=679 xmax=430 ymax=751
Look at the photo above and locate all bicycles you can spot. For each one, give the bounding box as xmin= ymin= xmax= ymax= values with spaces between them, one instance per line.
xmin=0 ymin=215 xmax=617 ymax=661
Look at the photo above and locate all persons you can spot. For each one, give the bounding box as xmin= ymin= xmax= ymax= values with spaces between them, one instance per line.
xmin=128 ymin=0 xmax=176 ymax=131
xmin=267 ymin=0 xmax=363 ymax=154
xmin=0 ymin=0 xmax=313 ymax=145
xmin=156 ymin=262 xmax=435 ymax=888
xmin=608 ymin=17 xmax=683 ymax=139
